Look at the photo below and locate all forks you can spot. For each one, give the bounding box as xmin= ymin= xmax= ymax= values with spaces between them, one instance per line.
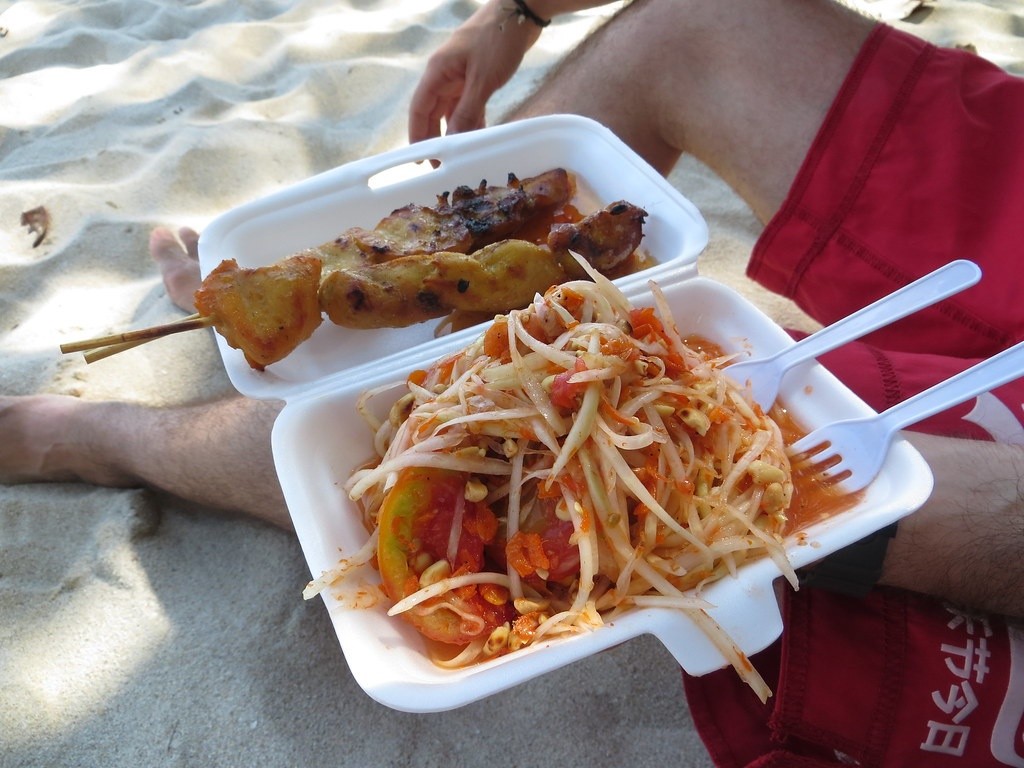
xmin=721 ymin=259 xmax=982 ymax=416
xmin=787 ymin=340 xmax=1024 ymax=496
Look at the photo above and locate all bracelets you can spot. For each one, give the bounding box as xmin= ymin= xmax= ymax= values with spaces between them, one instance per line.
xmin=784 ymin=518 xmax=898 ymax=600
xmin=500 ymin=0 xmax=551 ymax=34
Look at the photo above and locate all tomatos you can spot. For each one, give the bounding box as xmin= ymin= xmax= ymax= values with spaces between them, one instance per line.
xmin=630 ymin=309 xmax=686 ymax=374
xmin=498 ymin=481 xmax=581 ymax=583
xmin=377 ymin=467 xmax=510 ymax=643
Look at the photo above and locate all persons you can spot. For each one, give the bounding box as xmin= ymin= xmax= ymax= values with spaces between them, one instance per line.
xmin=0 ymin=0 xmax=1024 ymax=768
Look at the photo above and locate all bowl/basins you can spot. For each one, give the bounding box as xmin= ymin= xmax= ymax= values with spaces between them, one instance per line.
xmin=199 ymin=116 xmax=935 ymax=714
